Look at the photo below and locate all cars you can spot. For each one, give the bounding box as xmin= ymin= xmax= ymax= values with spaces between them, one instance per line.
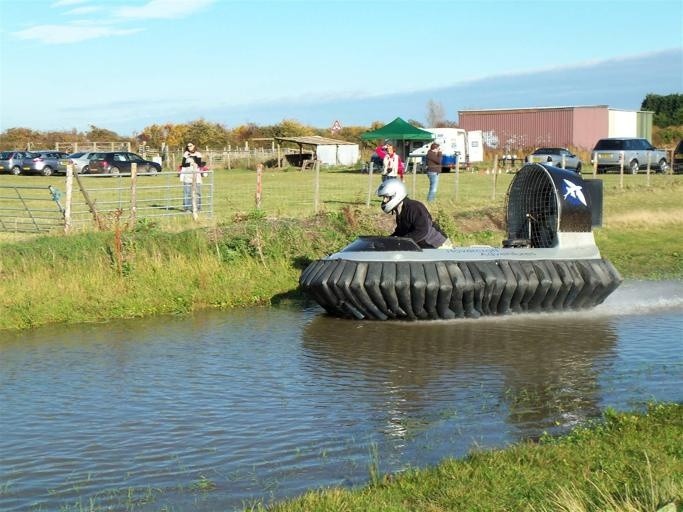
xmin=525 ymin=147 xmax=582 ymax=175
xmin=672 ymin=140 xmax=683 ymax=173
xmin=1 ymin=150 xmax=162 ymax=178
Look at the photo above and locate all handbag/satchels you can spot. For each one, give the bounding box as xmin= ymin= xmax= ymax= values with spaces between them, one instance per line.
xmin=180 ymin=167 xmax=202 ymax=184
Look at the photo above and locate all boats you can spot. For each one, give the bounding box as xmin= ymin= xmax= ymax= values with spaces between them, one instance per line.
xmin=300 ymin=164 xmax=624 ymax=322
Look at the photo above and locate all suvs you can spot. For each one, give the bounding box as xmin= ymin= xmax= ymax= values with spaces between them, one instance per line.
xmin=591 ymin=136 xmax=668 ymax=173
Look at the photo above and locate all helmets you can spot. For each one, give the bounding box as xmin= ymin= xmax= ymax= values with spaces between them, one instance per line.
xmin=376 ymin=178 xmax=408 ymax=215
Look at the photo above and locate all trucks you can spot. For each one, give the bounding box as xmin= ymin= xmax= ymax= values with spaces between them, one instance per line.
xmin=370 ymin=127 xmax=484 ymax=172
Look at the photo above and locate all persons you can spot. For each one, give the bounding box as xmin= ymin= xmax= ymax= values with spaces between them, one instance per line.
xmin=377 ymin=177 xmax=453 ymax=249
xmin=426 ymin=141 xmax=443 ymax=203
xmin=375 ymin=141 xmax=405 ymax=201
xmin=180 ymin=141 xmax=206 ymax=213
